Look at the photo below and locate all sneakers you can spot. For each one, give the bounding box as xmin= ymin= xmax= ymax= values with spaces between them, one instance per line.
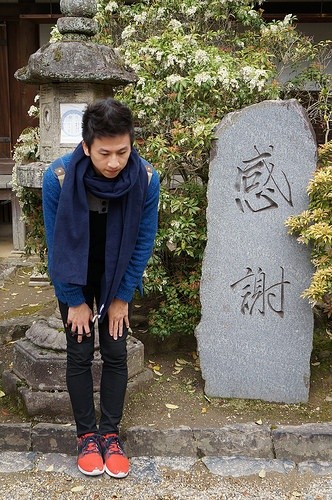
xmin=100 ymin=434 xmax=129 ymax=478
xmin=76 ymin=433 xmax=105 ymax=476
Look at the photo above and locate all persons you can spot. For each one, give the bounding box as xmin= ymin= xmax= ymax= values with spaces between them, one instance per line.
xmin=42 ymin=99 xmax=160 ymax=478
